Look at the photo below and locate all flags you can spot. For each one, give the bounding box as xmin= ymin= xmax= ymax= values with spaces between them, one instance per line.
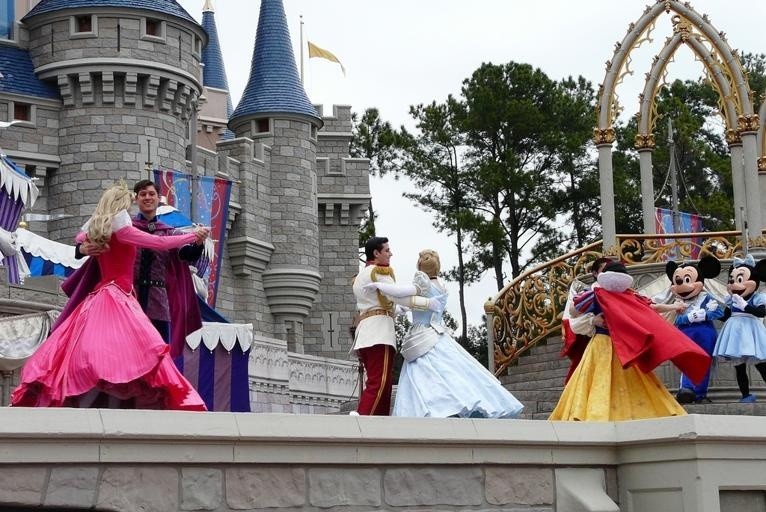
xmin=307 ymin=41 xmax=346 ymax=76
xmin=153 ymin=168 xmax=233 ymax=310
xmin=655 ymin=206 xmax=704 ymax=261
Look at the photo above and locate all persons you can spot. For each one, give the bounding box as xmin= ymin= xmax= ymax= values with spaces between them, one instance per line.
xmin=347 ymin=236 xmax=443 ymax=417
xmin=558 ymin=257 xmax=685 ymax=387
xmin=74 ymin=178 xmax=208 ymax=361
xmin=547 ymin=262 xmax=712 ymax=422
xmin=362 ymin=250 xmax=525 ymax=418
xmin=9 ymin=176 xmax=211 ymax=411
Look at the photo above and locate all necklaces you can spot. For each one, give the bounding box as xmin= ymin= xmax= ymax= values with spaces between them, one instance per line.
xmin=430 ymin=276 xmax=437 ymax=280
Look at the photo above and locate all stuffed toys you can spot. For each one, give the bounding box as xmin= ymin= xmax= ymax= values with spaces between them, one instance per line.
xmin=665 ymin=255 xmax=724 ymax=403
xmin=704 ymin=253 xmax=765 ymax=402
